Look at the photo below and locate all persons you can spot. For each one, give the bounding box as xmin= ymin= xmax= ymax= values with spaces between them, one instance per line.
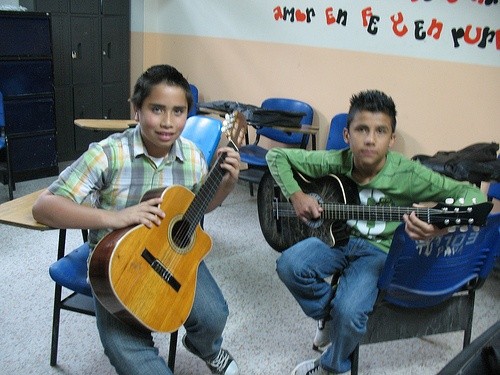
xmin=265 ymin=89 xmax=483 ymax=375
xmin=32 ymin=64 xmax=240 ymax=375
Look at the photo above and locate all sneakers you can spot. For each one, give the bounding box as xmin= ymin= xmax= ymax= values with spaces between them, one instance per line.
xmin=312 ymin=320 xmax=330 ymax=348
xmin=290 ymin=359 xmax=335 ymax=375
xmin=181 ymin=331 xmax=240 ymax=375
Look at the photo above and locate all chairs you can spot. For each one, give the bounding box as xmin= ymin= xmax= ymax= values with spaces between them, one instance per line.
xmin=0 ymin=86 xmax=500 ymax=375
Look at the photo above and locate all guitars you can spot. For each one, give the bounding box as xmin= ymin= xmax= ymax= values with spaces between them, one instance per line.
xmin=88 ymin=110 xmax=246 ymax=335
xmin=256 ymin=168 xmax=493 ymax=254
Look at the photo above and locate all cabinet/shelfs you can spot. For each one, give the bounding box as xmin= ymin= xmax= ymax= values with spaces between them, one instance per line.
xmin=0 ymin=0 xmax=131 ymax=200
